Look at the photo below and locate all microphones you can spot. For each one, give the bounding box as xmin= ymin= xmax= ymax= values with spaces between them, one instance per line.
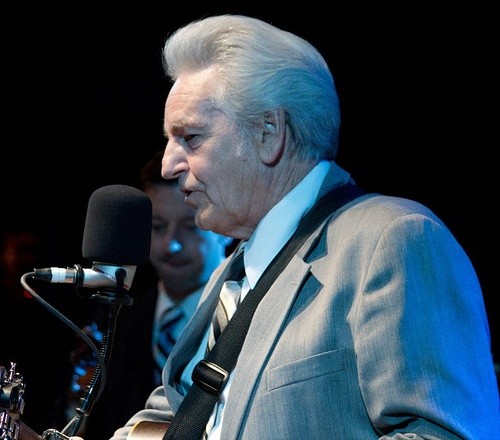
xmin=32 ymin=184 xmax=152 ymax=288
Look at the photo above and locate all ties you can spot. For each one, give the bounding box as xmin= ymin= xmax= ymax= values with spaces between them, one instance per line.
xmin=205 ymin=250 xmax=244 ymax=360
xmin=154 ymin=306 xmax=185 ymax=375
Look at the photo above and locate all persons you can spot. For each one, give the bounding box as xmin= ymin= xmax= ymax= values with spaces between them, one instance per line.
xmin=83 ymin=148 xmax=233 ymax=388
xmin=108 ymin=14 xmax=500 ymax=440
